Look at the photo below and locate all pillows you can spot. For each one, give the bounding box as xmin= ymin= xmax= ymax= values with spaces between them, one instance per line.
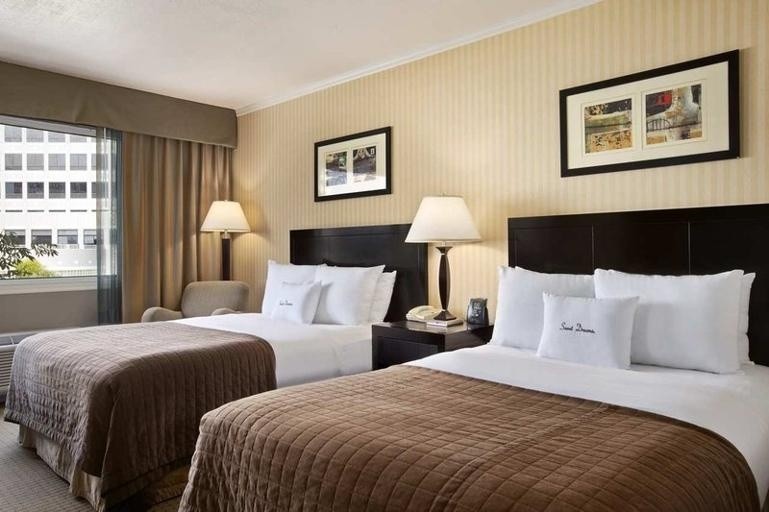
xmin=261 ymin=259 xmax=397 ymax=327
xmin=488 ymin=264 xmax=756 ymax=376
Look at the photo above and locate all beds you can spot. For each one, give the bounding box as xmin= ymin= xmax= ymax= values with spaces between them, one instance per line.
xmin=4 ymin=223 xmax=428 ymax=512
xmin=179 ymin=203 xmax=769 ymax=512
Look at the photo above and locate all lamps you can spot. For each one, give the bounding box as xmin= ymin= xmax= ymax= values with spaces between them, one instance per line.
xmin=200 ymin=199 xmax=251 ymax=281
xmin=405 ymin=197 xmax=482 ymax=321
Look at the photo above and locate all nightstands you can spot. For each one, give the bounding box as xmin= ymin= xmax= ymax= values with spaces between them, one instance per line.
xmin=372 ymin=320 xmax=494 ymax=371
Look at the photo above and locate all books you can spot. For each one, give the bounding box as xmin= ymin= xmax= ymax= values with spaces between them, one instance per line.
xmin=425 ymin=319 xmax=463 ymax=326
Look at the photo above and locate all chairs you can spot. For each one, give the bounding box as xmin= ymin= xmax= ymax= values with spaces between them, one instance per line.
xmin=140 ymin=280 xmax=249 ymax=323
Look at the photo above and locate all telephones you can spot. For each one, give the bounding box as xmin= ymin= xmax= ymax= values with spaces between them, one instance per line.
xmin=406 ymin=305 xmax=442 ymax=322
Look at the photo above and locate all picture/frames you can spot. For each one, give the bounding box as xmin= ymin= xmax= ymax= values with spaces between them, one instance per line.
xmin=313 ymin=126 xmax=391 ymax=201
xmin=560 ymin=49 xmax=739 ymax=179
